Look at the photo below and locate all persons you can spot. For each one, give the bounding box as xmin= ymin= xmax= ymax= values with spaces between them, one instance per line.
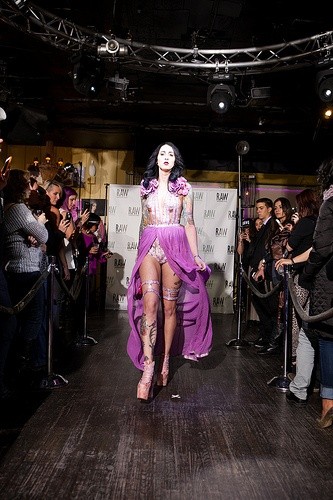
xmin=0 ymin=166 xmax=113 ymax=400
xmin=235 ymin=150 xmax=333 ymax=429
xmin=126 ymin=141 xmax=214 ymax=401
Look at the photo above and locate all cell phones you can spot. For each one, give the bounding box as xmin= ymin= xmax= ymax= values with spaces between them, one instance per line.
xmin=64 ymin=212 xmax=72 ymax=227
xmin=82 ymin=201 xmax=90 ymax=215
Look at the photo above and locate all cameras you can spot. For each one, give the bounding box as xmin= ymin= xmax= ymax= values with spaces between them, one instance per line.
xmin=241 ymin=228 xmax=245 ymax=234
